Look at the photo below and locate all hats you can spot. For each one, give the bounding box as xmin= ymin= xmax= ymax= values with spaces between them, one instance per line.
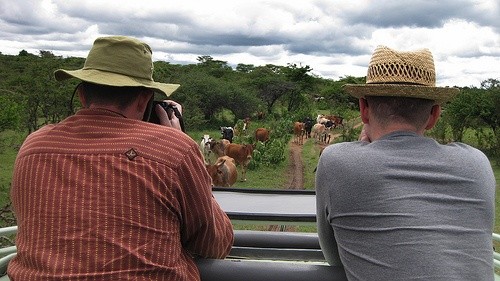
xmin=345 ymin=45 xmax=460 ymax=103
xmin=55 ymin=35 xmax=180 ymax=97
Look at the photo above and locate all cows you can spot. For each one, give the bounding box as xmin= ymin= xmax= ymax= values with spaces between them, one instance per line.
xmin=257 ymin=110 xmax=264 ymax=120
xmin=315 ymin=113 xmax=345 ymax=130
xmin=219 ymin=125 xmax=235 ymax=143
xmin=254 ymin=128 xmax=271 ymax=142
xmin=242 ymin=118 xmax=249 ymax=132
xmin=293 ymin=122 xmax=306 ymax=146
xmin=223 ymin=142 xmax=256 ymax=183
xmin=198 ymin=133 xmax=213 ymax=164
xmin=298 ymin=118 xmax=312 ymax=140
xmin=320 ymin=121 xmax=336 ymax=144
xmin=206 ymin=138 xmax=231 ymax=159
xmin=312 ymin=124 xmax=331 ymax=145
xmin=205 ymin=154 xmax=238 ymax=188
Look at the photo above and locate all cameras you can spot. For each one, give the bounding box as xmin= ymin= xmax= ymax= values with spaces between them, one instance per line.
xmin=142 ymin=100 xmax=171 ymax=124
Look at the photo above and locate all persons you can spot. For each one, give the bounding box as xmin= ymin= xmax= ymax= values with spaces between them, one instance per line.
xmin=315 ymin=47 xmax=496 ymax=280
xmin=7 ymin=36 xmax=235 ymax=281
